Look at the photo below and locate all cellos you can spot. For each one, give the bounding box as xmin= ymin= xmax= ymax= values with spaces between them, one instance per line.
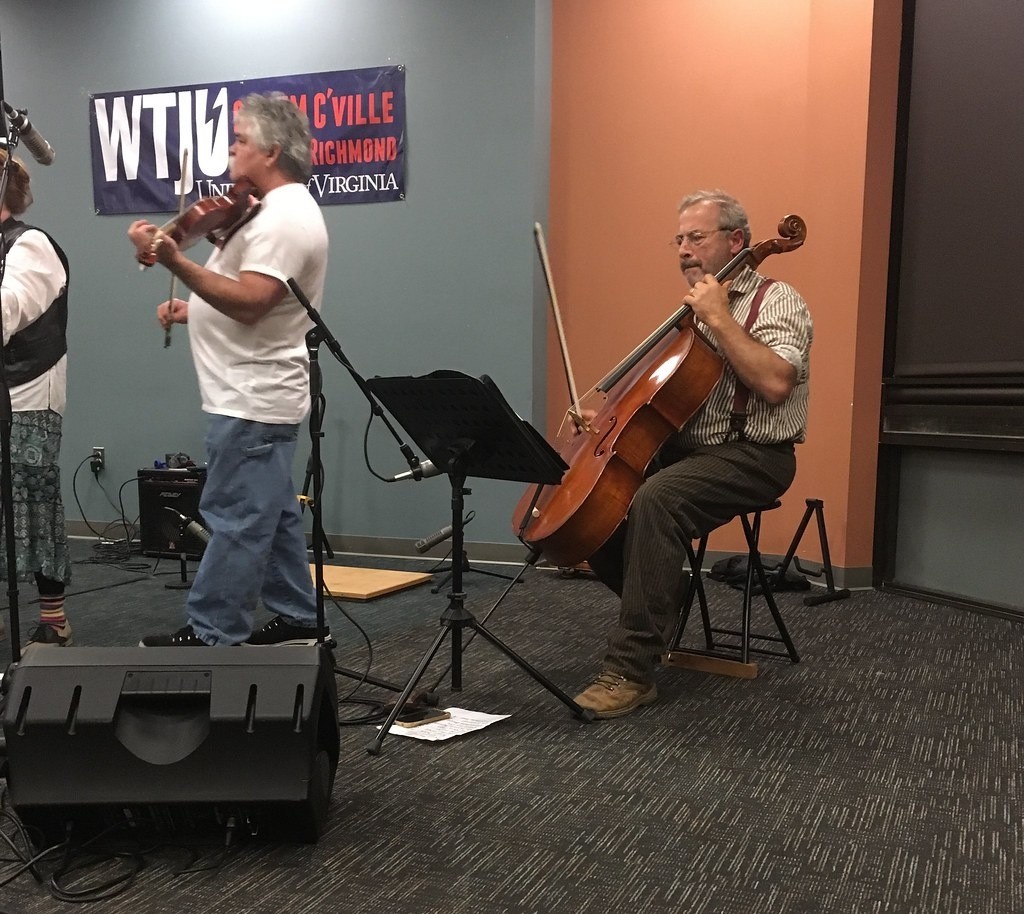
xmin=419 ymin=213 xmax=807 ymax=705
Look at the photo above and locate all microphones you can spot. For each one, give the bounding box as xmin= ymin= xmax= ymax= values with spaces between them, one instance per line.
xmin=188 ymin=520 xmax=212 ymax=548
xmin=7 ymin=112 xmax=56 ymax=166
xmin=411 ymin=458 xmax=443 ymax=479
xmin=415 ymin=525 xmax=454 ymax=553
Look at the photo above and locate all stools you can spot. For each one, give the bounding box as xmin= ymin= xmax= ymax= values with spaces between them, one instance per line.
xmin=660 ymin=500 xmax=801 ymax=678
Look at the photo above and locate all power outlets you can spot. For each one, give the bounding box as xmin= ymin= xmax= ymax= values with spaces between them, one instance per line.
xmin=93 ymin=447 xmax=105 ymax=468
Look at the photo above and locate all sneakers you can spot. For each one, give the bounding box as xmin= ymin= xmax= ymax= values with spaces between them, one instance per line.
xmin=242 ymin=615 xmax=331 ymax=646
xmin=25 ymin=619 xmax=73 ymax=649
xmin=572 ymin=670 xmax=661 ymax=721
xmin=138 ymin=625 xmax=211 ymax=647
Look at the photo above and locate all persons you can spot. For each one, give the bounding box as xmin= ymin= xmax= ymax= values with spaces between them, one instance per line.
xmin=0 ymin=146 xmax=71 ymax=657
xmin=573 ymin=185 xmax=814 ymax=721
xmin=128 ymin=91 xmax=335 ymax=646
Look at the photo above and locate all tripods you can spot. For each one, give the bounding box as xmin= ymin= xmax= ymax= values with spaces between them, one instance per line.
xmin=420 ymin=551 xmax=525 ymax=594
xmin=0 ymin=127 xmax=31 ymax=717
xmin=286 ymin=278 xmax=442 ymax=709
xmin=365 ymin=370 xmax=596 ymax=756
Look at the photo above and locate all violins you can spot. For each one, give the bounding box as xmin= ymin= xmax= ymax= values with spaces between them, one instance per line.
xmin=136 ymin=175 xmax=254 ymax=267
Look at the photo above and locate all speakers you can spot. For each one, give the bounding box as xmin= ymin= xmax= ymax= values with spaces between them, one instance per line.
xmin=139 ymin=468 xmax=207 ymax=561
xmin=0 ymin=645 xmax=341 ymax=849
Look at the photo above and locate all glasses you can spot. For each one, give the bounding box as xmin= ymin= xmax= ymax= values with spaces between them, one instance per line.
xmin=673 ymin=227 xmax=728 ymax=249
